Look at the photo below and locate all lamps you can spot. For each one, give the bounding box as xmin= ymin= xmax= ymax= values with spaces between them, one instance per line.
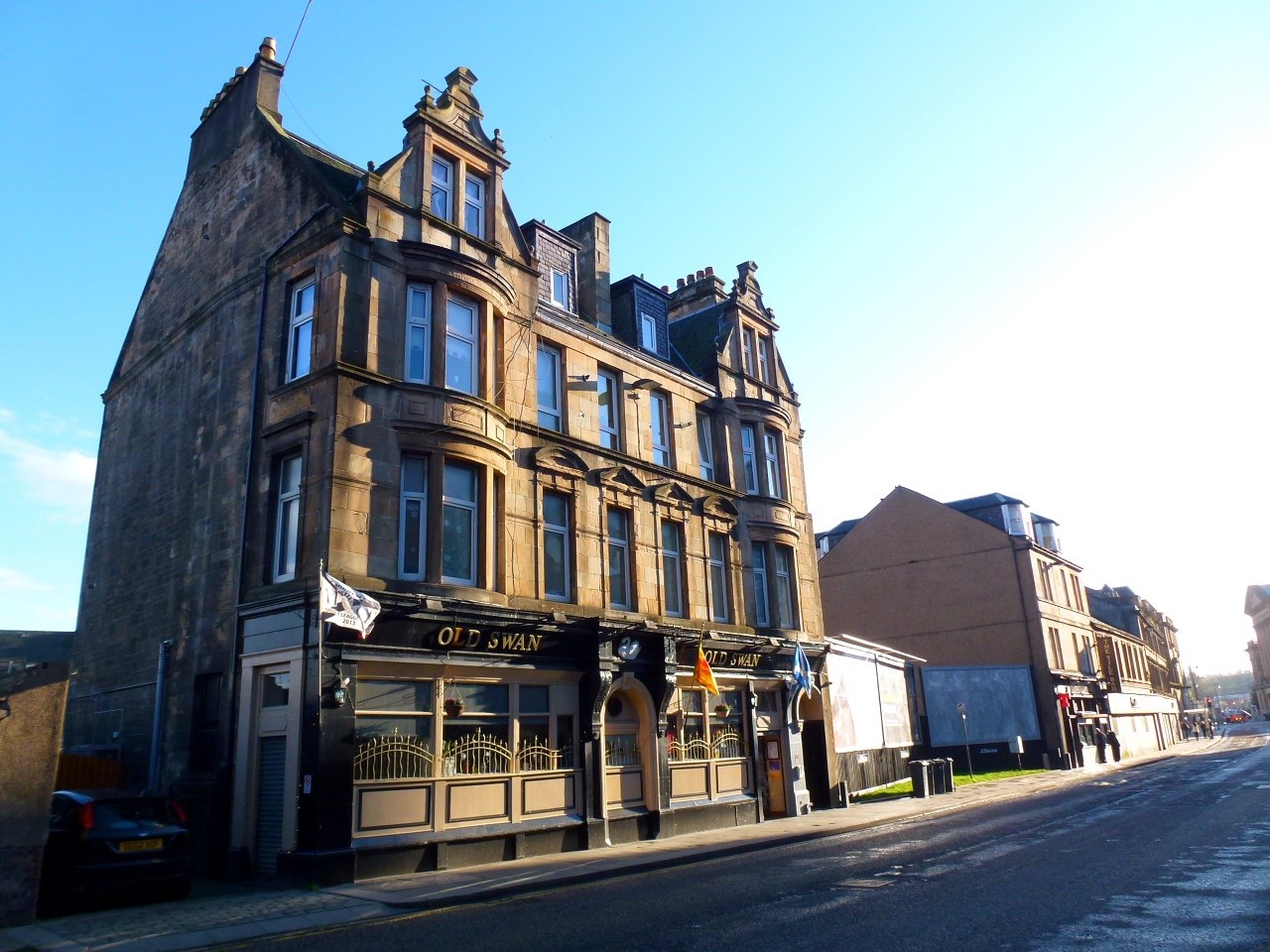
xmin=534 ymin=611 xmax=568 ymax=627
xmin=703 ymin=632 xmax=720 ymax=639
xmin=632 ymin=379 xmax=663 ymax=390
xmin=637 ymin=619 xmax=658 ymax=631
xmin=765 ymin=638 xmax=781 ymax=646
xmin=332 ymin=675 xmax=348 ymax=708
xmin=746 ymin=691 xmax=758 ymax=709
xmin=416 ymin=599 xmax=443 ymax=613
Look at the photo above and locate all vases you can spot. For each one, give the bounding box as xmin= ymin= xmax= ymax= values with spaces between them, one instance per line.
xmin=716 ymin=711 xmax=730 ymax=717
xmin=444 ymin=706 xmax=464 ymax=718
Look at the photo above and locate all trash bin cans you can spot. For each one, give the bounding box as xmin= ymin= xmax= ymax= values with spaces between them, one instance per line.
xmin=926 ymin=760 xmax=936 ymax=795
xmin=944 ymin=758 xmax=956 ymax=792
xmin=909 ymin=760 xmax=930 ymax=799
xmin=931 ymin=758 xmax=946 ymax=794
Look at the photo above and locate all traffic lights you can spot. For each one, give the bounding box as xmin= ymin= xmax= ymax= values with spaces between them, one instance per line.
xmin=1207 ymin=698 xmax=1212 ymax=707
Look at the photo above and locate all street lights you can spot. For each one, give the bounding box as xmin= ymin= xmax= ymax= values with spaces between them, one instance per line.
xmin=1214 ymin=683 xmax=1221 ymax=708
xmin=1189 ymin=670 xmax=1199 ymax=708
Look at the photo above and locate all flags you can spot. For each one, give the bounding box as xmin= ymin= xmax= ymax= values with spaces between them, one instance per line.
xmin=792 ymin=644 xmax=811 ymax=700
xmin=319 ymin=568 xmax=381 ymax=639
xmin=694 ymin=643 xmax=720 ymax=696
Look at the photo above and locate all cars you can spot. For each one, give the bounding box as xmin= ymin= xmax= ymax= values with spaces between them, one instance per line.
xmin=42 ymin=791 xmax=190 ymax=904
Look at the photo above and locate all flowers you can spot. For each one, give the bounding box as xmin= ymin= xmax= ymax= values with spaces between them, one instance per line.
xmin=445 ymin=699 xmax=464 ymax=705
xmin=714 ymin=703 xmax=730 ymax=711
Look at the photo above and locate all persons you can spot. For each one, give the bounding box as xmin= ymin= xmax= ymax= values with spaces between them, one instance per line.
xmin=1096 ymin=727 xmax=1121 ymax=764
xmin=1182 ymin=718 xmax=1215 ymax=740
